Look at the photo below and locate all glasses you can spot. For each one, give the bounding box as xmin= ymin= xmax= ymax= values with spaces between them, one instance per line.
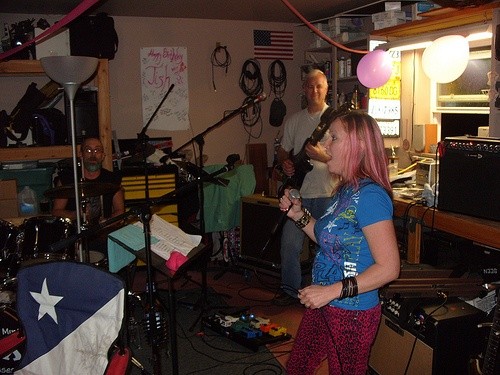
xmin=82 ymin=148 xmax=103 ymax=153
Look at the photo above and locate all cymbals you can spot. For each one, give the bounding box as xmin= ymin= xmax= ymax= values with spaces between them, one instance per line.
xmin=42 ymin=180 xmax=115 ymax=199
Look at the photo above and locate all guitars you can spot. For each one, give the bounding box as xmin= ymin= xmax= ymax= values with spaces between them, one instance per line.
xmin=279 ymin=101 xmax=355 ymax=191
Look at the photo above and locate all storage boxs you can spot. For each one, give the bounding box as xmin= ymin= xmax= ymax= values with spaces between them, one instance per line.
xmin=312 ymin=0 xmax=437 ymax=47
xmin=0 ymin=166 xmax=53 ymax=217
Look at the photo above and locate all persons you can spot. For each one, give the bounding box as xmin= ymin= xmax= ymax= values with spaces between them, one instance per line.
xmin=279 ymin=110 xmax=400 ymax=375
xmin=274 ymin=69 xmax=342 ymax=304
xmin=53 ymin=137 xmax=125 ymax=228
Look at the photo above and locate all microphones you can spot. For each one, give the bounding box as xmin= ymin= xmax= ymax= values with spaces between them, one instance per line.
xmin=242 ymin=92 xmax=268 ymax=108
xmin=270 ymin=189 xmax=302 ymax=237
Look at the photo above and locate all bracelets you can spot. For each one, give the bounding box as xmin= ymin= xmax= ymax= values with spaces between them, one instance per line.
xmin=338 ymin=276 xmax=358 ymax=302
xmin=295 ymin=208 xmax=312 ymax=228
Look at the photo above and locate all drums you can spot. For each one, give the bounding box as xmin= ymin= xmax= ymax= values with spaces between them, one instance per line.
xmin=0 ymin=303 xmax=28 ymax=375
xmin=0 ymin=219 xmax=23 ymax=287
xmin=17 ymin=214 xmax=77 ymax=266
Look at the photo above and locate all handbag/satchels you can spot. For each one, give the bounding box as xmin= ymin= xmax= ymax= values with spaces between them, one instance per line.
xmin=70 ymin=12 xmax=119 ymax=60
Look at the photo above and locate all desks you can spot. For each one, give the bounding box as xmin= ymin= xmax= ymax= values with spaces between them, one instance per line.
xmin=393 ymin=187 xmax=500 ymax=268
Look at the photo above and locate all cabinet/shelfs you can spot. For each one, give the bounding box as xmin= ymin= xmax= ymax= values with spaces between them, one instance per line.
xmin=0 ymin=57 xmax=113 ymax=225
xmin=296 ymin=36 xmax=385 ymax=115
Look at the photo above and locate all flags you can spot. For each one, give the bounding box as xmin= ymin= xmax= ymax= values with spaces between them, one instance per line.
xmin=253 ymin=29 xmax=295 ymax=61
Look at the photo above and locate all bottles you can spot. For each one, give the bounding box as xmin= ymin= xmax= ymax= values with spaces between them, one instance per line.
xmin=17 ymin=186 xmax=39 ymax=217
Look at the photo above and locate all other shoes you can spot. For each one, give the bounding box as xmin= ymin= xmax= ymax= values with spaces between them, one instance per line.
xmin=272 ymin=292 xmax=300 ymax=306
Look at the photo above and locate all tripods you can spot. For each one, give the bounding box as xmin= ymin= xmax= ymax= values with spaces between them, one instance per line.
xmin=160 ymin=105 xmax=246 ymax=332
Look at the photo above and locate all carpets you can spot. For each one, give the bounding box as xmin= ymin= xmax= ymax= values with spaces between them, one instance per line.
xmin=117 ymin=286 xmax=289 ymax=375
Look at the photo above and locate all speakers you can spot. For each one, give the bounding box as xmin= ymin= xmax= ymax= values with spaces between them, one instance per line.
xmin=436 ymin=135 xmax=500 ymax=222
xmin=369 ymin=299 xmax=481 ymax=373
xmin=239 ymin=193 xmax=310 ymax=267
xmin=64 ymin=90 xmax=98 ymax=145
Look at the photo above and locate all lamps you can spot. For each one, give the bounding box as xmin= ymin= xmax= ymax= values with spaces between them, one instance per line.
xmin=388 ymin=24 xmax=494 ymax=52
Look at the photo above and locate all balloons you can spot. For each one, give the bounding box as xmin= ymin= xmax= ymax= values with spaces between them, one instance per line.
xmin=422 ymin=34 xmax=470 ymax=84
xmin=357 ymin=49 xmax=394 ymax=88
